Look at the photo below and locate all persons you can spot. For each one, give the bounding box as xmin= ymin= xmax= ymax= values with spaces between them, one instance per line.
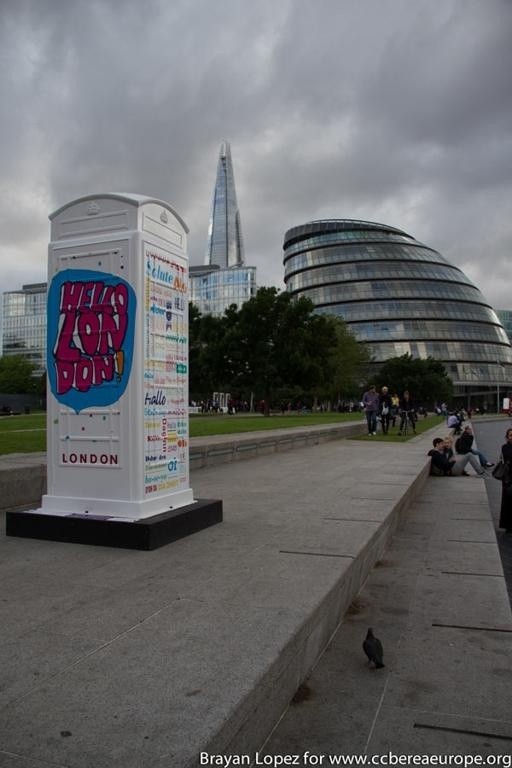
xmin=316 ymin=384 xmax=417 ymax=436
xmin=189 ymin=397 xmax=308 ymax=416
xmin=3 ymin=404 xmax=13 ymax=416
xmin=419 ymin=400 xmax=495 ymax=477
xmin=499 ymin=428 xmax=512 ymax=533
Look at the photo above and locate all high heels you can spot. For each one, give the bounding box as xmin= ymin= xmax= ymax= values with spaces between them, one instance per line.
xmin=482 ymin=463 xmax=494 ymax=469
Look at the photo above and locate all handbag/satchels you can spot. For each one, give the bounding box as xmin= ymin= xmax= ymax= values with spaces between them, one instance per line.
xmin=382 ymin=401 xmax=389 ymax=415
xmin=492 ymin=444 xmax=512 ymax=480
